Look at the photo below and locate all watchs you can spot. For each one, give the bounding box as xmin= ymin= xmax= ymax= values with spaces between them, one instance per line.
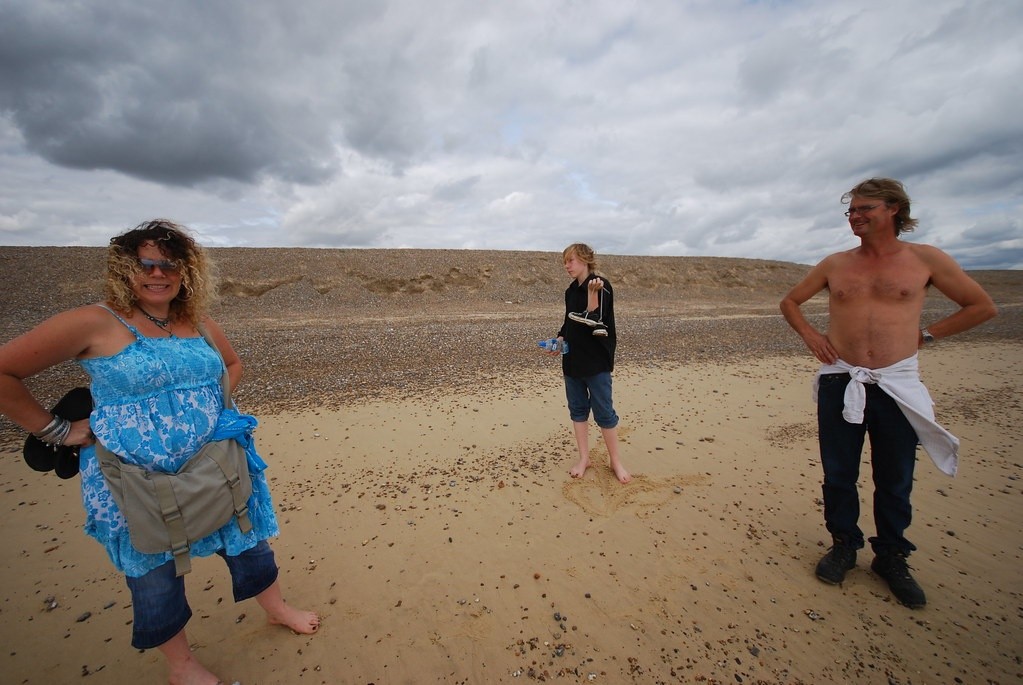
xmin=920 ymin=327 xmax=935 ymax=347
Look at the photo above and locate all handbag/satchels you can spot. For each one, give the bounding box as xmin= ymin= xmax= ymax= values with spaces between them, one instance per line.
xmin=98 ymin=440 xmax=253 ymax=582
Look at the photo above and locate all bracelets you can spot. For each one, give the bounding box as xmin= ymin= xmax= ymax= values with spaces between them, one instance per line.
xmin=31 ymin=414 xmax=72 ymax=452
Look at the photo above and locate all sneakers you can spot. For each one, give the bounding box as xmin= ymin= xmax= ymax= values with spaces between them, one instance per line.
xmin=815 ymin=539 xmax=859 ymax=585
xmin=869 ymin=551 xmax=927 ymax=610
xmin=568 ymin=311 xmax=599 ymax=327
xmin=592 ymin=323 xmax=609 ymax=337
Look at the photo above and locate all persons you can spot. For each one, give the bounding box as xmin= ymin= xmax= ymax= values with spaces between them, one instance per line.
xmin=0 ymin=218 xmax=320 ymax=685
xmin=780 ymin=176 xmax=998 ymax=611
xmin=549 ymin=244 xmax=631 ymax=485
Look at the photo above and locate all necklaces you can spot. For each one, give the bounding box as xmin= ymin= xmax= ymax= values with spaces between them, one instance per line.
xmin=133 ymin=301 xmax=180 ymax=341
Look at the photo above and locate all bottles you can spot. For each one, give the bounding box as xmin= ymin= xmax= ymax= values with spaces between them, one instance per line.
xmin=539 ymin=339 xmax=569 ymax=355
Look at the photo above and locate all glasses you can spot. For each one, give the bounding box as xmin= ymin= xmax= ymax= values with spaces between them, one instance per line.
xmin=138 ymin=258 xmax=180 ymax=277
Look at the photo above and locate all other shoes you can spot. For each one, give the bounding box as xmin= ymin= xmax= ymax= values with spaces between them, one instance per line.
xmin=22 ymin=386 xmax=94 ymax=480
